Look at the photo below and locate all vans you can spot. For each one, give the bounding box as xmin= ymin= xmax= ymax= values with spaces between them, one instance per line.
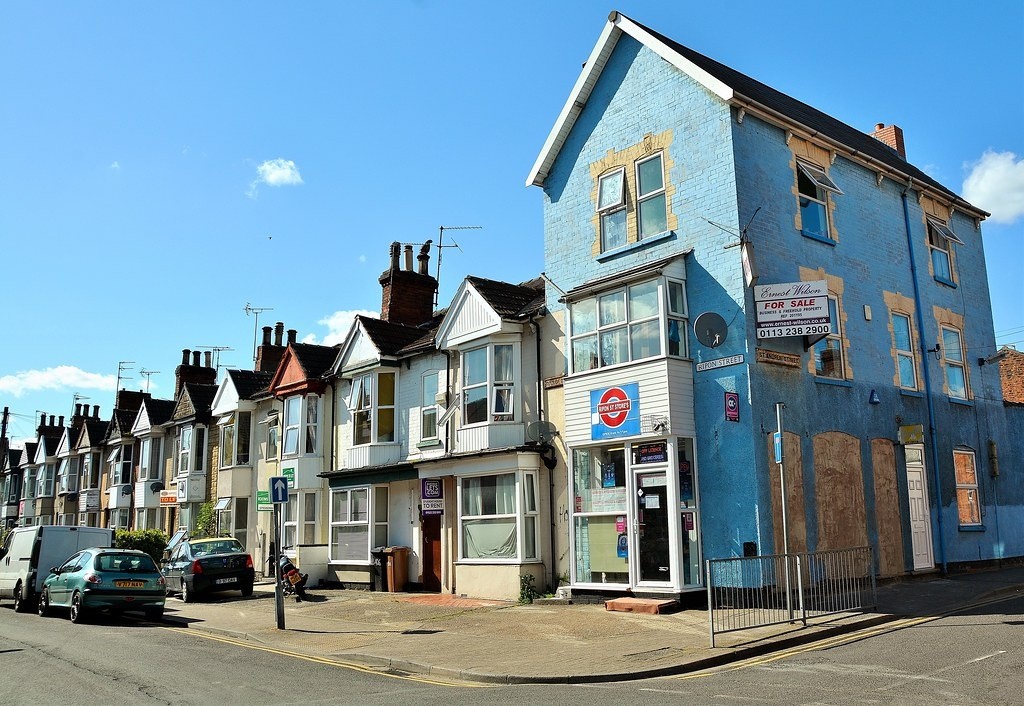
xmin=0 ymin=524 xmax=113 ymax=613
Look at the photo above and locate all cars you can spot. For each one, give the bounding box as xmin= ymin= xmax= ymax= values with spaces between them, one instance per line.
xmin=160 ymin=537 xmax=254 ymax=604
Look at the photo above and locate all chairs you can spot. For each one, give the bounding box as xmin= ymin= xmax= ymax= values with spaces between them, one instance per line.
xmin=119 ymin=560 xmax=133 ymax=571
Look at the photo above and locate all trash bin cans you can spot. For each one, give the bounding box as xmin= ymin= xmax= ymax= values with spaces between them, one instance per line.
xmin=382 ymin=546 xmax=411 ymax=592
xmin=370 ymin=546 xmax=388 ymax=592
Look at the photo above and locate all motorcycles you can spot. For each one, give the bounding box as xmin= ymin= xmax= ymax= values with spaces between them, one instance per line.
xmin=265 ymin=544 xmax=309 ymax=601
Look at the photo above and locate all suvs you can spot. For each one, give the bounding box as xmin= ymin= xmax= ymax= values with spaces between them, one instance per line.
xmin=38 ymin=546 xmax=166 ymax=624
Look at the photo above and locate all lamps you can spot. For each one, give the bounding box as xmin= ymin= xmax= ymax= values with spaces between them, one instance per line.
xmin=979 ymin=352 xmax=1005 ymax=367
